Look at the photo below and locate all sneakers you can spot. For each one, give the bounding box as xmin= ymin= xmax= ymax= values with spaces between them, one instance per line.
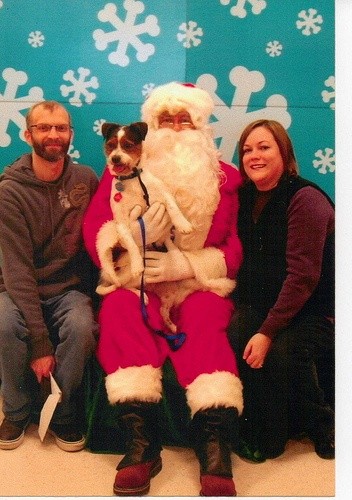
xmin=264 ymin=433 xmax=287 ymax=458
xmin=199 ymin=473 xmax=237 ymax=497
xmin=47 ymin=423 xmax=85 ymax=451
xmin=0 ymin=415 xmax=31 ymax=449
xmin=113 ymin=454 xmax=162 ymax=496
xmin=315 ymin=434 xmax=335 ymax=460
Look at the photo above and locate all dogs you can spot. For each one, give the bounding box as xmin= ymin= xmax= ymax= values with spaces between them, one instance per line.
xmin=97 ymin=120 xmax=212 ymax=334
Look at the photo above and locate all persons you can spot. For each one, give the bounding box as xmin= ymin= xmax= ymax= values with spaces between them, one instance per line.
xmin=81 ymin=81 xmax=242 ymax=496
xmin=232 ymin=120 xmax=339 ymax=464
xmin=1 ymin=100 xmax=102 ymax=453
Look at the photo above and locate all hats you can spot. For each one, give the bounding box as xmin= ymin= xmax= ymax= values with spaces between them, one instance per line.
xmin=141 ymin=82 xmax=214 ymax=129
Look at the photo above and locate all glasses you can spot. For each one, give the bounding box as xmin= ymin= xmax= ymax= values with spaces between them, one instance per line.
xmin=159 ymin=122 xmax=194 ymax=129
xmin=28 ymin=123 xmax=69 ymax=132
